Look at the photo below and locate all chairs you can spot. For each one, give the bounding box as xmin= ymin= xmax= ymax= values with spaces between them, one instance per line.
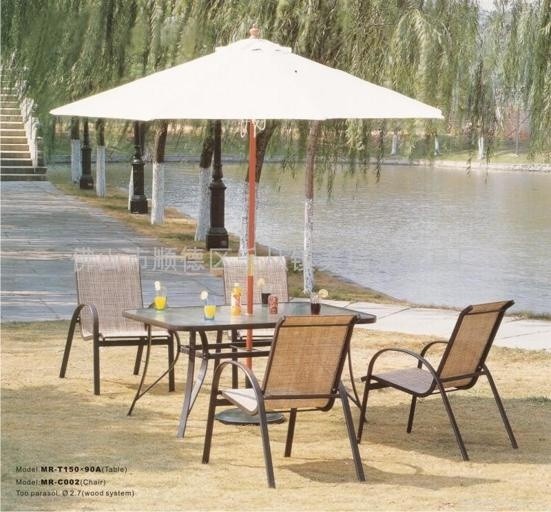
xmin=357 ymin=299 xmax=518 ymax=460
xmin=59 ymin=253 xmax=175 ymax=395
xmin=221 ymin=255 xmax=288 ymax=388
xmin=201 ymin=314 xmax=365 ymax=488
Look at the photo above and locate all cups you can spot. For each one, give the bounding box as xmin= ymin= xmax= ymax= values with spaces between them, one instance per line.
xmin=153 ymin=287 xmax=167 ymax=311
xmin=261 ymin=283 xmax=274 ymax=307
xmin=310 ymin=292 xmax=322 ymax=314
xmin=202 ymin=295 xmax=217 ymax=321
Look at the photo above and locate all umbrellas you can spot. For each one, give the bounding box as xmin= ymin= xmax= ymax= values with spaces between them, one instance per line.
xmin=48 ymin=29 xmax=446 ymax=388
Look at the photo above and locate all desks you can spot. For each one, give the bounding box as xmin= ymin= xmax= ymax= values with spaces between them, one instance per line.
xmin=123 ymin=302 xmax=376 ymax=439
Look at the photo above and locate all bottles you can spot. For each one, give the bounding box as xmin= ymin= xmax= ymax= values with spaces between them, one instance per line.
xmin=268 ymin=295 xmax=278 ymax=316
xmin=231 ymin=282 xmax=242 ymax=316
